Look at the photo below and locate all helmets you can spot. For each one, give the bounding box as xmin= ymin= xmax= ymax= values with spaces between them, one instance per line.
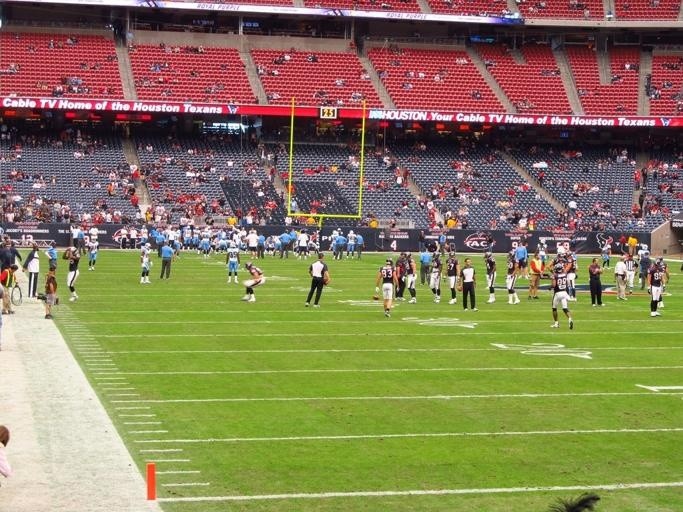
xmin=385 ymin=251 xmax=412 ymax=265
xmin=507 ymin=250 xmax=516 ymax=259
xmin=230 ymin=242 xmax=237 ymax=248
xmin=654 ymin=256 xmax=664 ymax=265
xmin=484 ymin=251 xmax=492 ymax=259
xmin=553 ymin=250 xmax=574 ymax=271
xmin=144 ymin=242 xmax=152 ymax=249
xmin=70 ymin=247 xmax=78 ymax=256
xmin=90 ymin=236 xmax=97 ymax=244
xmin=434 ymin=252 xmax=456 ymax=260
xmin=246 ymin=261 xmax=253 ymax=270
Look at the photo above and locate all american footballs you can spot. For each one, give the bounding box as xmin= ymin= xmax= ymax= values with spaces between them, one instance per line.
xmin=373 ymin=294 xmax=379 ymax=300
xmin=324 ymin=278 xmax=328 ymax=285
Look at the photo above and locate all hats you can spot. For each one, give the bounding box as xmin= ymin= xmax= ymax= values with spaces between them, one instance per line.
xmin=629 ymin=245 xmax=649 ymax=258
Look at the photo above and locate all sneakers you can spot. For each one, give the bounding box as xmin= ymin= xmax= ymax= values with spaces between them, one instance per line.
xmin=305 ymin=303 xmax=321 ymax=308
xmin=507 ymin=299 xmax=520 ymax=305
xmin=464 ymin=307 xmax=479 ymax=312
xmin=528 ymin=295 xmax=540 ymax=300
xmin=617 ymin=290 xmax=633 ymax=301
xmin=487 ymin=298 xmax=495 ymax=303
xmin=139 ymin=280 xmax=153 ymax=284
xmin=592 ymin=303 xmax=606 ymax=308
xmin=45 ymin=313 xmax=53 ymax=319
xmin=88 ymin=266 xmax=96 ymax=272
xmin=244 ymin=249 xmax=362 ymax=260
xmin=515 ymin=272 xmax=531 ymax=279
xmin=650 ymin=301 xmax=665 ymax=317
xmin=568 ymin=296 xmax=577 ymax=301
xmin=384 ymin=311 xmax=390 ymax=318
xmin=549 ymin=323 xmax=560 ymax=328
xmin=227 ymin=280 xmax=239 ymax=284
xmin=396 ymin=296 xmax=417 ymax=303
xmin=174 ymin=247 xmax=225 ymax=259
xmin=568 ymin=320 xmax=573 ymax=329
xmin=241 ymin=297 xmax=256 ymax=302
xmin=433 ymin=297 xmax=441 ymax=303
xmin=69 ymin=295 xmax=79 ymax=302
xmin=449 ymin=298 xmax=457 ymax=304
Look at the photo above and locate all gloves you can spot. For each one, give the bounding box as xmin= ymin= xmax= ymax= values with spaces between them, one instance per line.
xmin=647 ymin=288 xmax=652 ymax=294
xmin=662 ymin=286 xmax=666 ymax=292
xmin=375 ymin=286 xmax=380 ymax=293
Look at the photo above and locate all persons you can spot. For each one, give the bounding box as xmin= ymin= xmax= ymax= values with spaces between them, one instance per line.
xmin=0 ymin=1 xmax=682 ymax=330
xmin=0 ymin=425 xmax=10 ymax=477
xmin=546 ymin=492 xmax=601 ymax=512
